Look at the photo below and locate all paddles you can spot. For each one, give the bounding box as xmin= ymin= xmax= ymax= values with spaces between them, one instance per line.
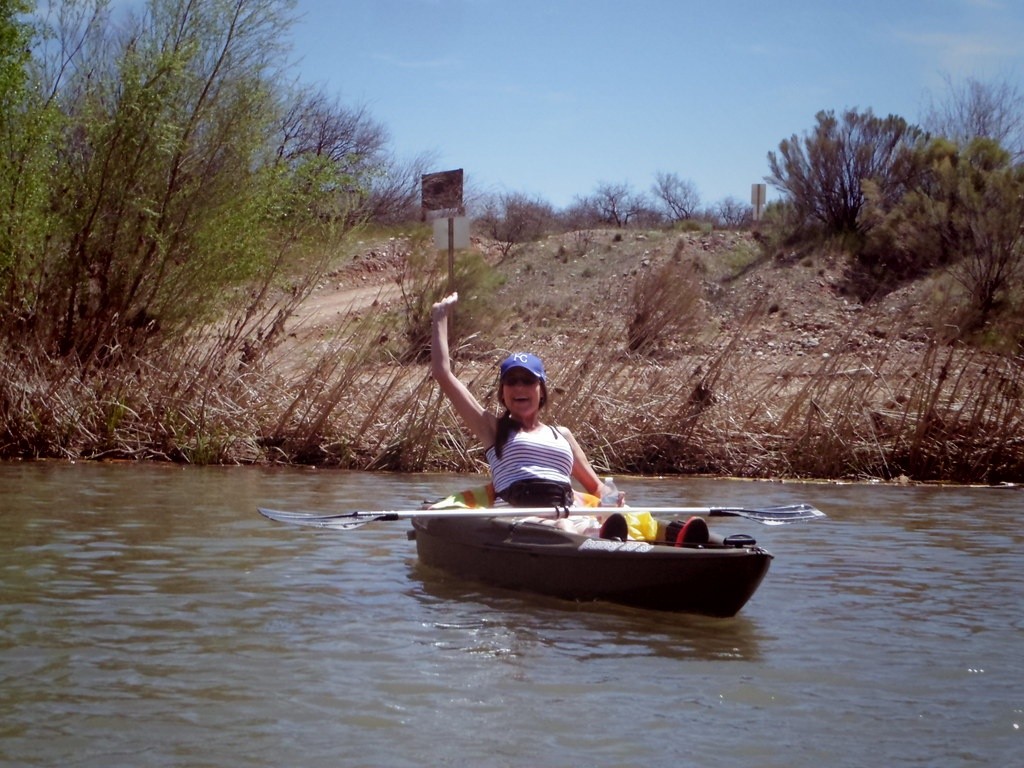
xmin=256 ymin=504 xmax=826 ymax=531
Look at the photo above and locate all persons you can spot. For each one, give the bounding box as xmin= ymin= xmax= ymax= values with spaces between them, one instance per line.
xmin=430 ymin=291 xmax=710 ymax=548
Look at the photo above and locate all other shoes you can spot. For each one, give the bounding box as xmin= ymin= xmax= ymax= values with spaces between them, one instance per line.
xmin=674 ymin=516 xmax=710 ymax=548
xmin=600 ymin=513 xmax=628 ymax=541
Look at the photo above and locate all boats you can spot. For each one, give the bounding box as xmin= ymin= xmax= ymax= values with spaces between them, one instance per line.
xmin=406 ymin=484 xmax=774 ymax=618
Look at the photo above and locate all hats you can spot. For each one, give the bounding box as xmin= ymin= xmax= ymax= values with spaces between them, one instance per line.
xmin=500 ymin=353 xmax=547 ymax=383
